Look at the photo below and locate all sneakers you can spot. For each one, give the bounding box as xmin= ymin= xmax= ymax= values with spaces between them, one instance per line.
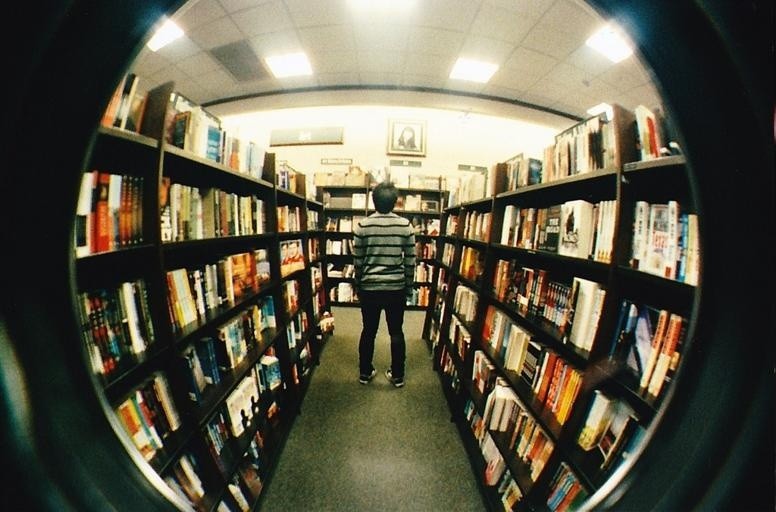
xmin=359 ymin=368 xmax=378 ymax=384
xmin=384 ymin=368 xmax=405 ymax=388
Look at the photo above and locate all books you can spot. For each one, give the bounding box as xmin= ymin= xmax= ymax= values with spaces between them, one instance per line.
xmin=323 ymin=192 xmax=375 ymax=304
xmin=73 ymin=73 xmax=325 ymax=512
xmin=392 ymin=194 xmax=440 ymax=307
xmin=430 ymin=103 xmax=700 ymax=512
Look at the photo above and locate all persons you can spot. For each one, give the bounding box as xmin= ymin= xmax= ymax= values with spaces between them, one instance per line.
xmin=352 ymin=181 xmax=417 ymax=389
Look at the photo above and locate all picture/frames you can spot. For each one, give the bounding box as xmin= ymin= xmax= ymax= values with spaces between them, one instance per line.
xmin=387 ymin=117 xmax=427 ymax=157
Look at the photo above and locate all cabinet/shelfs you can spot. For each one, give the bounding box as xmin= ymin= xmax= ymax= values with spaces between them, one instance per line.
xmin=421 ymin=104 xmax=699 ymax=510
xmin=306 ymin=171 xmax=450 ymax=312
xmin=74 ymin=86 xmax=332 ymax=512
